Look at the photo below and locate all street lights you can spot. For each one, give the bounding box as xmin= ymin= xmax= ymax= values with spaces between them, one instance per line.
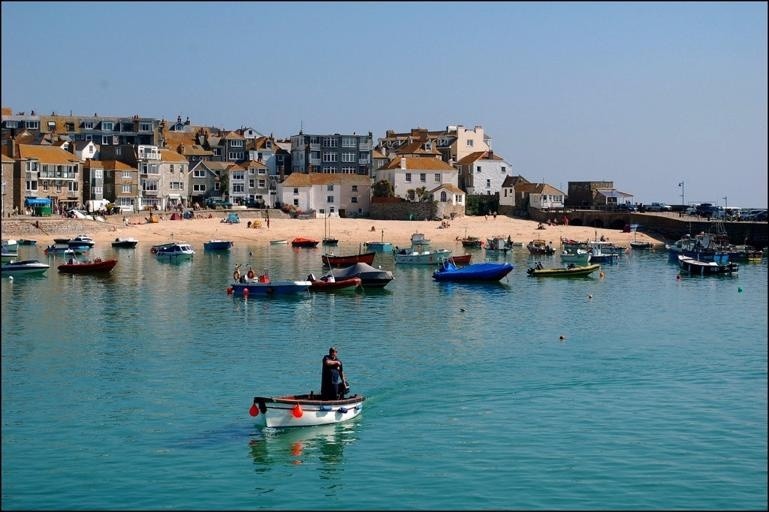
xmin=678 ymin=179 xmax=684 ymax=205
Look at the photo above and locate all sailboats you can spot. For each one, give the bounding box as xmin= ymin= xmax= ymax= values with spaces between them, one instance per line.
xmin=323 ymin=208 xmax=339 ymax=246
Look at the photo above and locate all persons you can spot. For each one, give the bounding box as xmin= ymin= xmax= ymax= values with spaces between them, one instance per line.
xmin=310 ymin=274 xmax=315 ymax=280
xmin=69 ymin=259 xmax=72 ymax=264
xmin=539 ymin=262 xmax=542 ymax=268
xmin=320 ymin=347 xmax=346 ymax=401
xmin=245 ymin=271 xmax=254 ymax=283
xmin=326 ymin=275 xmax=335 ymax=282
xmin=485 ymin=209 xmax=496 ymax=219
xmin=258 ymin=275 xmax=268 ymax=283
xmin=538 ymin=216 xmax=568 ymax=230
xmin=535 ymin=263 xmax=541 ymax=269
xmin=508 ymin=235 xmax=512 ymax=242
xmin=601 ymin=235 xmax=605 ymax=241
xmin=547 ymin=241 xmax=552 ymax=255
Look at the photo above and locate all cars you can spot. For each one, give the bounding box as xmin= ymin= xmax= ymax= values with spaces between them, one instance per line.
xmin=687 ymin=203 xmax=768 ymax=220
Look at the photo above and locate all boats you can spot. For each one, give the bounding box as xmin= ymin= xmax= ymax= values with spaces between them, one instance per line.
xmin=231 ymin=264 xmax=313 ymax=297
xmin=526 ymin=263 xmax=601 ymax=277
xmin=56 ymin=257 xmax=118 ymax=274
xmin=45 ymin=233 xmax=95 ymax=255
xmin=526 ymin=235 xmax=557 ymax=255
xmin=319 ymin=262 xmax=395 ymax=288
xmin=150 ymin=242 xmax=195 ymax=259
xmin=448 ymin=254 xmax=472 ymax=264
xmin=630 ymin=231 xmax=656 ymax=250
xmin=461 ymin=227 xmax=485 ymax=250
xmin=665 ymin=231 xmax=769 ymax=276
xmin=291 ymin=237 xmax=319 ymax=248
xmin=252 ymin=391 xmax=368 ymax=430
xmin=1 ymin=259 xmax=50 ymax=275
xmin=560 ymin=230 xmax=628 ymax=263
xmin=269 ymin=240 xmax=288 ymax=245
xmin=486 ymin=236 xmax=524 ymax=250
xmin=366 ymin=229 xmax=393 ymax=253
xmin=203 ymin=241 xmax=234 ymax=251
xmin=112 ymin=238 xmax=138 ymax=248
xmin=392 ymin=229 xmax=453 ymax=265
xmin=1 ymin=239 xmax=37 ymax=257
xmin=432 ymin=262 xmax=514 ymax=282
xmin=306 ymin=255 xmax=361 ymax=292
xmin=321 ymin=243 xmax=377 ymax=268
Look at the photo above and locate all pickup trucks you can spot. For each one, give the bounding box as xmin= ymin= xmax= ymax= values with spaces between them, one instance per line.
xmin=644 ymin=200 xmax=673 ymax=212
